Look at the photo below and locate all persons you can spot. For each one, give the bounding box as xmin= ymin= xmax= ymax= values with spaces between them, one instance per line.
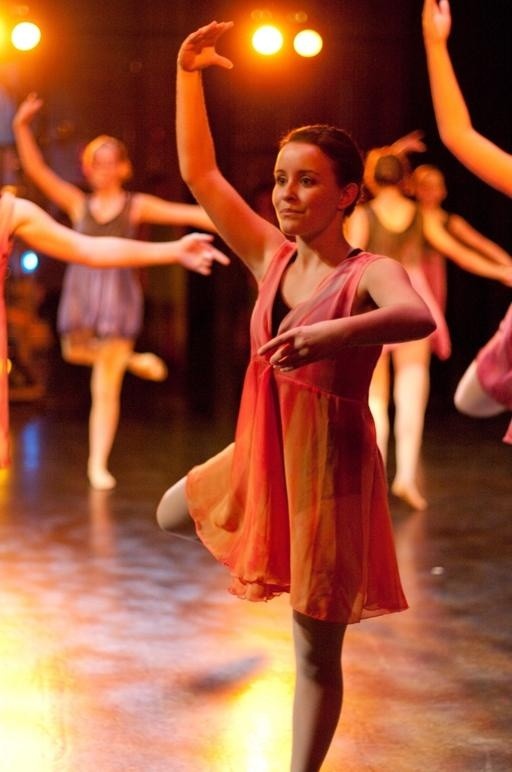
xmin=4 ymin=303 xmax=54 ymax=404
xmin=365 ymin=131 xmax=510 ymax=313
xmin=12 ymin=88 xmax=220 ymax=491
xmin=344 ymin=159 xmax=510 ymax=511
xmin=0 ymin=188 xmax=231 ymax=469
xmin=420 ymin=1 xmax=512 ymax=449
xmin=155 ymin=19 xmax=436 ymax=769
